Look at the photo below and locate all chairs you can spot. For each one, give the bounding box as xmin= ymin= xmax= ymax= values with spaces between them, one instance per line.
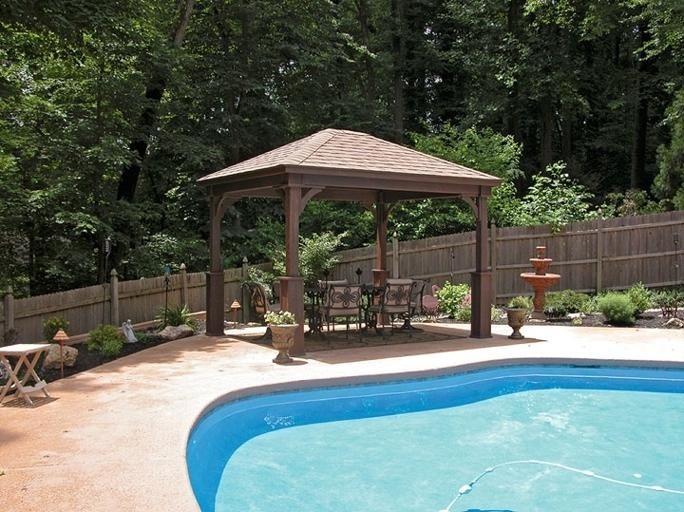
xmin=240 ymin=278 xmax=427 ymax=346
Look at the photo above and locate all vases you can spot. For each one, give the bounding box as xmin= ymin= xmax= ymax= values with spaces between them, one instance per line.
xmin=507 ymin=310 xmax=528 ymax=339
xmin=267 ymin=323 xmax=300 ymax=364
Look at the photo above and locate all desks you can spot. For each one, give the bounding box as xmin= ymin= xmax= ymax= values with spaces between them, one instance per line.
xmin=0 ymin=344 xmax=52 ymax=407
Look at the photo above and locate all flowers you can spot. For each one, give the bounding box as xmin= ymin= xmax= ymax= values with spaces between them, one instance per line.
xmin=264 ymin=310 xmax=296 ymax=325
xmin=507 ymin=297 xmax=530 ymax=309
